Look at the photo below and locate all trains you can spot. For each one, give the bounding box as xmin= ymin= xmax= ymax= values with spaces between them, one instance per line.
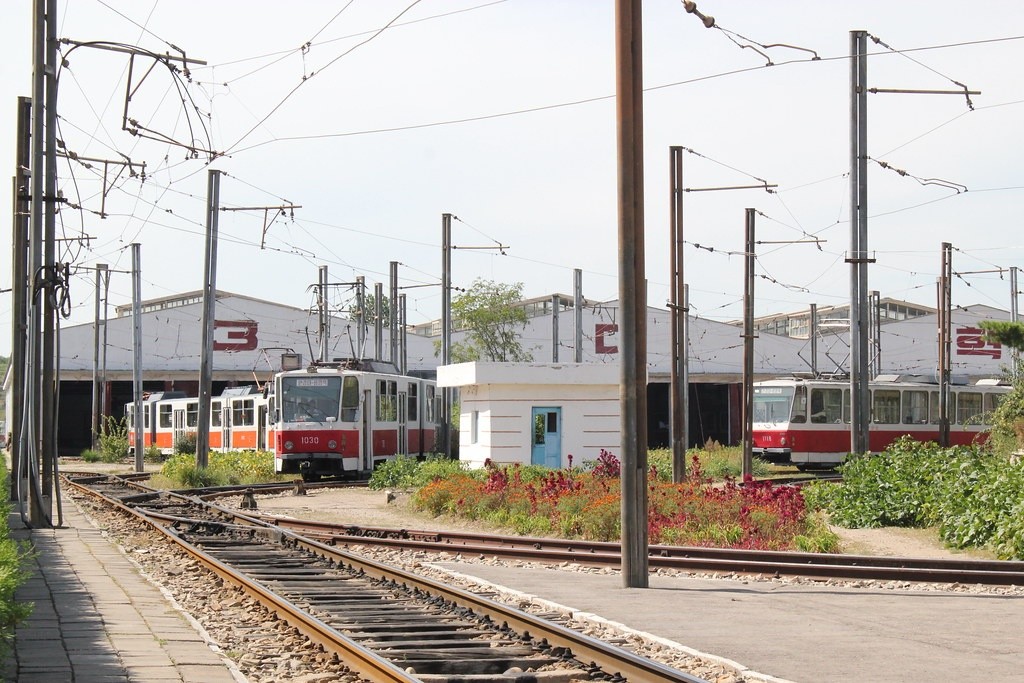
xmin=123 ymin=324 xmax=444 ymax=484
xmin=747 ymin=317 xmax=1014 ymax=472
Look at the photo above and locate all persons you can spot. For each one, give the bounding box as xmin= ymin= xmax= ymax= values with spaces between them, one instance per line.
xmin=296 ymin=399 xmax=324 ymax=414
